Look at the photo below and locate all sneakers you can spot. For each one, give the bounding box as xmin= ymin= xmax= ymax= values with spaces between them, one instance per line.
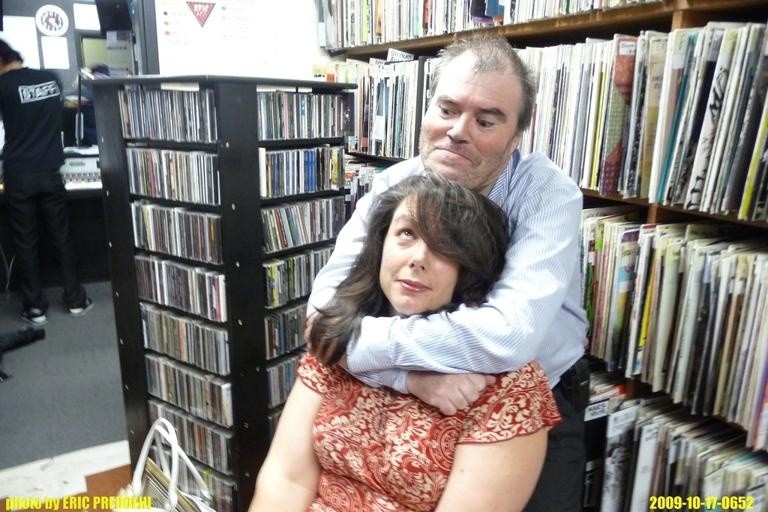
xmin=16 ymin=307 xmax=49 ymax=328
xmin=68 ymin=297 xmax=95 ymax=317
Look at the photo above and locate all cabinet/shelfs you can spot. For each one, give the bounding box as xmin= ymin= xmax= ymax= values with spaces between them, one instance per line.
xmin=78 ymin=75 xmax=358 ymax=512
xmin=344 ymin=1 xmax=768 ymax=397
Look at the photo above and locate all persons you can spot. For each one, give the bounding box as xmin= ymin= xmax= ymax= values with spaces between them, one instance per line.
xmin=0 ymin=40 xmax=94 ymax=327
